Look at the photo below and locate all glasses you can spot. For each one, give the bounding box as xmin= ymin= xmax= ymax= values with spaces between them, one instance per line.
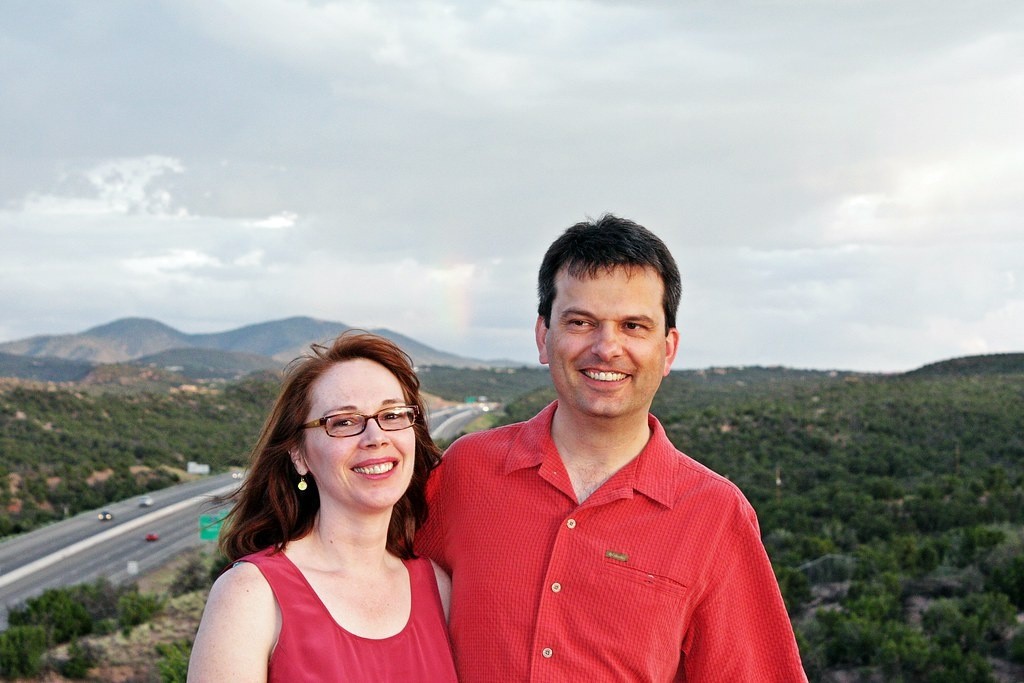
xmin=300 ymin=404 xmax=421 ymax=437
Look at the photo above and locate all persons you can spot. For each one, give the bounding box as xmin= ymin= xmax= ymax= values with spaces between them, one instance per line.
xmin=186 ymin=335 xmax=453 ymax=683
xmin=411 ymin=216 xmax=809 ymax=683
